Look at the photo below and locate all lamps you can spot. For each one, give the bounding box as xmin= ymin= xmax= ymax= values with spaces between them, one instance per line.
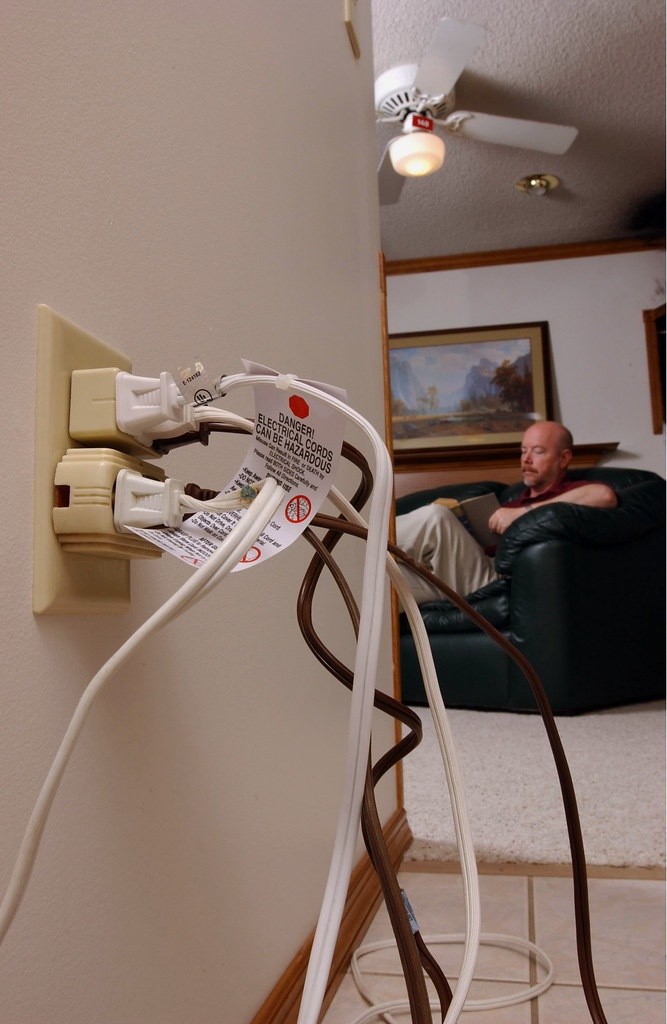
xmin=515 ymin=171 xmax=562 ymax=198
xmin=382 ymin=111 xmax=447 ymax=178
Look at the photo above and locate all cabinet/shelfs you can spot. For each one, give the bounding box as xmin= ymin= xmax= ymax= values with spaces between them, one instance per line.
xmin=392 ymin=440 xmax=622 ymax=502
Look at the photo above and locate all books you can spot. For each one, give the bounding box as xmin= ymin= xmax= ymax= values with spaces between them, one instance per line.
xmin=431 ymin=492 xmax=501 ymax=543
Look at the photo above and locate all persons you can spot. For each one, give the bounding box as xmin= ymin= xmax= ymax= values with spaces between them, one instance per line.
xmin=394 ymin=421 xmax=617 ymax=615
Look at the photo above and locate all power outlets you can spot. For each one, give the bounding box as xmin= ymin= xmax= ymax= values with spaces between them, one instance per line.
xmin=27 ymin=295 xmax=178 ymax=628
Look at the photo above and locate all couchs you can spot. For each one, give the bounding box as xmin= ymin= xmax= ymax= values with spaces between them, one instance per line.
xmin=392 ymin=451 xmax=666 ymax=720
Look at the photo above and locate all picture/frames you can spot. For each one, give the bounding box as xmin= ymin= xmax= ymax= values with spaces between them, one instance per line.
xmin=388 ymin=317 xmax=562 ymax=458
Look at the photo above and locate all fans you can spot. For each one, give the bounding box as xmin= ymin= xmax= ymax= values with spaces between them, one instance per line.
xmin=375 ymin=11 xmax=587 ymax=222
